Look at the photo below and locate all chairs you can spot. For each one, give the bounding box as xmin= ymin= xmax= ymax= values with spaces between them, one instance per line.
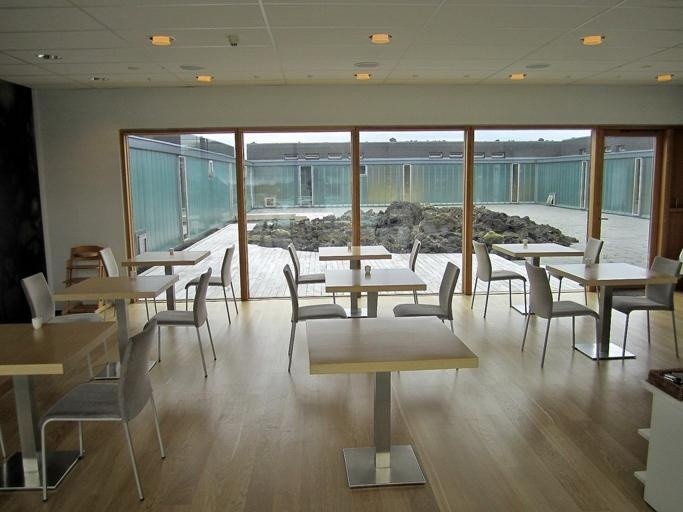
xmin=546 ymin=237 xmax=605 ymax=305
xmin=60 ymin=245 xmax=113 ymax=315
xmin=142 ymin=267 xmax=216 ymax=378
xmin=22 ymin=273 xmax=108 ymax=380
xmin=611 ymin=256 xmax=682 ymax=361
xmin=521 ymin=261 xmax=601 ymax=369
xmin=394 ymin=262 xmax=460 ymax=374
xmin=409 ymin=238 xmax=422 ymax=304
xmin=185 ymin=244 xmax=238 ymax=325
xmin=471 ymin=239 xmax=528 ymax=318
xmin=287 ymin=243 xmax=340 ymax=304
xmin=283 ymin=264 xmax=348 ymax=374
xmin=99 ymin=247 xmax=157 ymax=321
xmin=37 ymin=314 xmax=166 ymax=503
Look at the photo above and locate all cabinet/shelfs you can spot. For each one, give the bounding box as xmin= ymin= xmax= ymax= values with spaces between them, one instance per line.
xmin=634 ymin=369 xmax=682 ymax=509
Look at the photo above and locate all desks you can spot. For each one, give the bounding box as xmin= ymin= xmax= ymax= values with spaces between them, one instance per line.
xmin=306 ymin=316 xmax=480 ymax=488
xmin=1 ymin=321 xmax=119 ymax=490
xmin=122 ymin=251 xmax=211 ymax=311
xmin=546 ymin=262 xmax=677 ymax=360
xmin=492 ymin=243 xmax=585 ymax=315
xmin=51 ymin=275 xmax=179 ymax=381
xmin=326 ymin=267 xmax=428 ymax=318
xmin=318 ymin=246 xmax=392 ymax=317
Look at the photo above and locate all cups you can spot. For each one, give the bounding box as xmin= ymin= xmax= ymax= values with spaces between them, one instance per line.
xmin=585 ymin=258 xmax=591 ymax=267
xmin=31 ymin=317 xmax=41 ymax=330
xmin=347 ymin=242 xmax=351 ymax=249
xmin=523 ymin=239 xmax=527 ymax=247
xmin=364 ymin=266 xmax=371 ymax=274
xmin=168 ymin=248 xmax=173 ymax=255
